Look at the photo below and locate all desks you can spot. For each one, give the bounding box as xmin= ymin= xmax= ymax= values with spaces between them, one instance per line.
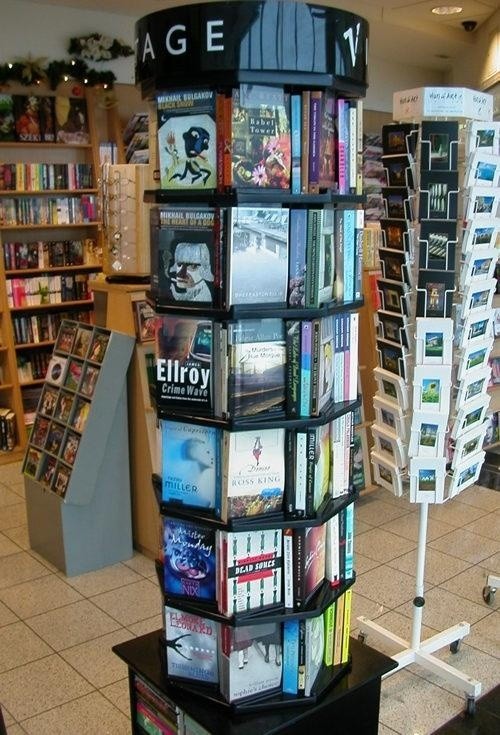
xmin=112 ymin=618 xmax=398 ymax=735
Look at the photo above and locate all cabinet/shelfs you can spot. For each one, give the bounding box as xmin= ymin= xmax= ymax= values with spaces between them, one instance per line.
xmin=352 ymin=81 xmax=497 ymax=714
xmin=140 ymin=0 xmax=376 ymax=715
xmin=96 ymin=266 xmax=383 ymax=566
xmin=23 ymin=320 xmax=139 ymax=576
xmin=1 ymin=86 xmax=108 ymax=467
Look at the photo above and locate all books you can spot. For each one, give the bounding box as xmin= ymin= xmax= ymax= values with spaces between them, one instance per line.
xmin=370 ymin=119 xmax=500 ymax=503
xmin=132 ymin=674 xmax=208 ymax=735
xmin=1 ymin=94 xmax=91 ymax=144
xmin=1 ymin=386 xmax=43 ymax=452
xmin=1 ymin=162 xmax=102 ymax=227
xmin=23 ymin=325 xmax=109 ymax=499
xmin=0 ymin=238 xmax=98 ymax=380
xmin=133 ymin=204 xmax=365 ymax=422
xmin=123 ymin=85 xmax=363 ymax=197
xmin=156 ymin=410 xmax=355 ymax=706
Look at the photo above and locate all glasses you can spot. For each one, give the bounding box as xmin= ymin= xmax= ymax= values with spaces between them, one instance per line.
xmin=96 ymin=176 xmax=142 ymax=269
